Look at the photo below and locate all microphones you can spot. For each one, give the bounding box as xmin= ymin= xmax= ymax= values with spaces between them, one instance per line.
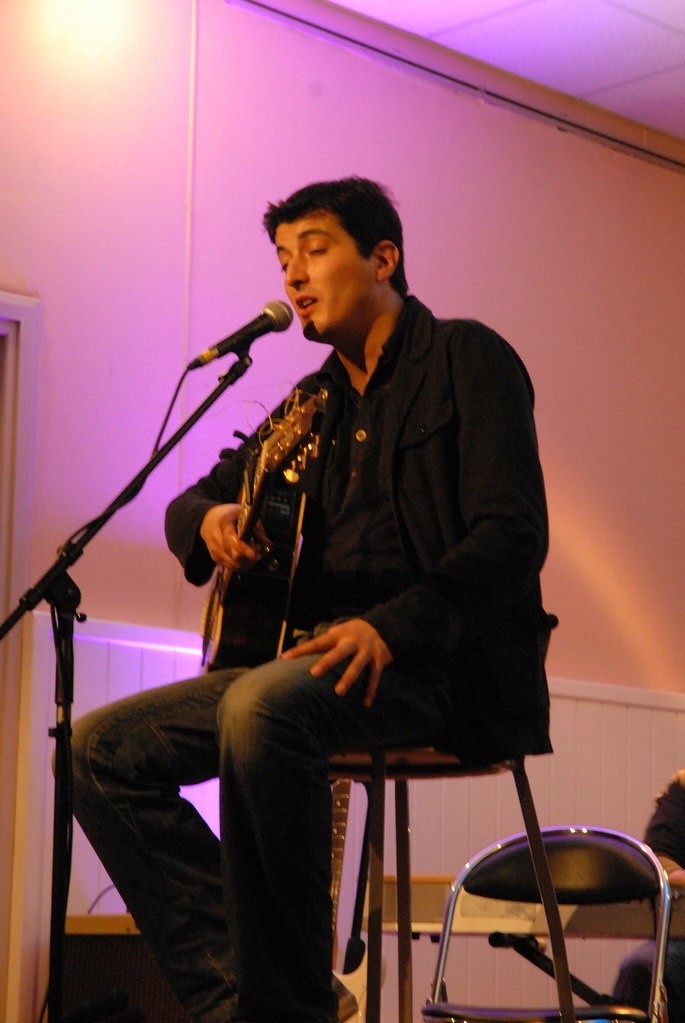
xmin=184 ymin=301 xmax=294 ymax=371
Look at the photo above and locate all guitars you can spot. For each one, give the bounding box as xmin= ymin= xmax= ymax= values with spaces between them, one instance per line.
xmin=200 ymin=378 xmax=330 ymax=669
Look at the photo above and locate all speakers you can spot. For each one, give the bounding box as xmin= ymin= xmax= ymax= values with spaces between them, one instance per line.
xmin=60 ymin=914 xmax=193 ymax=1023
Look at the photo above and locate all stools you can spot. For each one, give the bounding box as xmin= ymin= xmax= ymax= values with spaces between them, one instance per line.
xmin=326 ymin=744 xmax=580 ymax=1022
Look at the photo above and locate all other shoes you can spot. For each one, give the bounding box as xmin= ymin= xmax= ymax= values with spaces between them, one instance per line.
xmin=193 ymin=973 xmax=359 ymax=1023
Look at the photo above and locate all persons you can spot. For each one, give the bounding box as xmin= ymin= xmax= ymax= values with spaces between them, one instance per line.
xmin=612 ymin=766 xmax=685 ymax=1023
xmin=50 ymin=174 xmax=550 ymax=1023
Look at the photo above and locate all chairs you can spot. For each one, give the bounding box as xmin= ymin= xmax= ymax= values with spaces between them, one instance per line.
xmin=421 ymin=825 xmax=672 ymax=1023
